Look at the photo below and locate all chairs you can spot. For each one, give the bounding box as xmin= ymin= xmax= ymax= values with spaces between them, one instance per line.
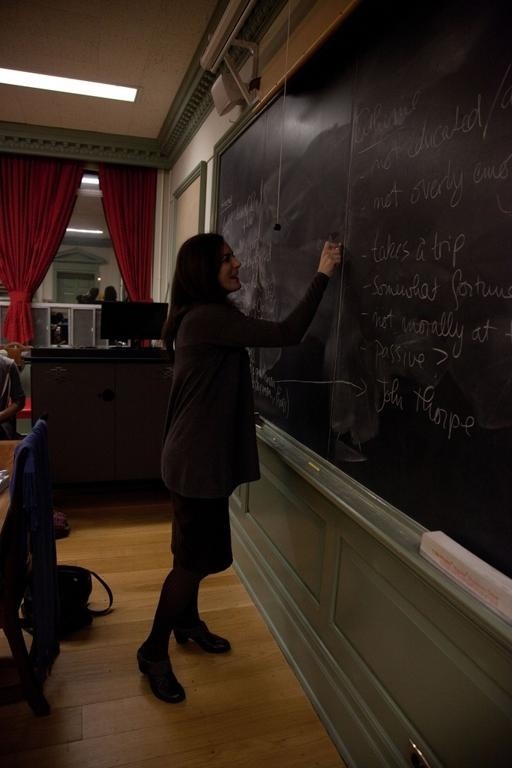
xmin=1 ymin=419 xmax=74 ymax=717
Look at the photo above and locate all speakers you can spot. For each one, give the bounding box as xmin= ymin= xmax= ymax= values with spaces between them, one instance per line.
xmin=210 ymin=72 xmax=248 ymax=116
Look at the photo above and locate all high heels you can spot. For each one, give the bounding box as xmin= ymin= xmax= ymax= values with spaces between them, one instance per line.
xmin=174 ymin=620 xmax=230 ymax=653
xmin=136 ymin=647 xmax=185 ymax=704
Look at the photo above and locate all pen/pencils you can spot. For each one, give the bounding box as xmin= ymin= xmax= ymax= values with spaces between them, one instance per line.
xmin=308 ymin=462 xmax=321 ymax=472
xmin=256 ymin=424 xmax=263 ymax=430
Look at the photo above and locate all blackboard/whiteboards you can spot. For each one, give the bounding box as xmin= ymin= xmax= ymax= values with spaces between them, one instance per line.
xmin=208 ymin=1 xmax=512 ymax=651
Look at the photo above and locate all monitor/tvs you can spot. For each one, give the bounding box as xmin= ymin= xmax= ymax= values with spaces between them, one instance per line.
xmin=100 ymin=302 xmax=169 ymax=350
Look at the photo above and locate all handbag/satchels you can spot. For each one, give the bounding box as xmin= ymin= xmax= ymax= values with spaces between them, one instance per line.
xmin=22 ymin=566 xmax=91 ymax=640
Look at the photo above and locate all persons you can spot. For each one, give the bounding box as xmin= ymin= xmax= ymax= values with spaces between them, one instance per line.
xmin=137 ymin=230 xmax=340 ymax=703
xmin=0 ymin=353 xmax=26 ymax=440
